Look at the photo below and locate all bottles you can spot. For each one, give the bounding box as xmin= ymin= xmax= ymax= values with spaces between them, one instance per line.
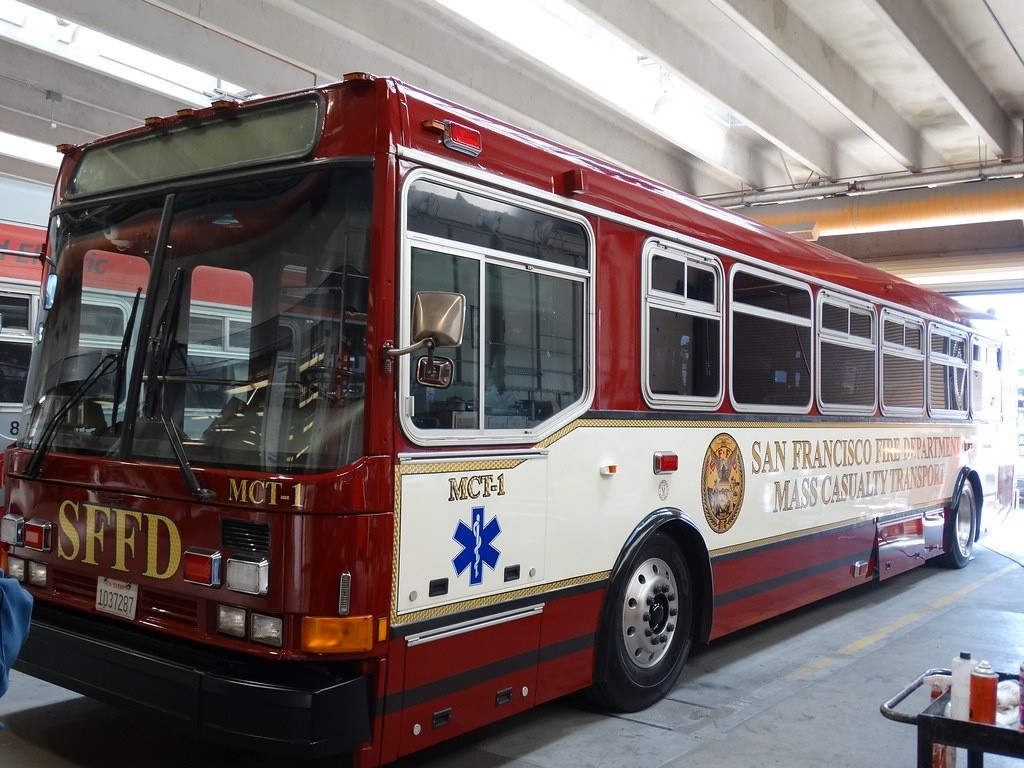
xmin=951 ymin=651 xmax=978 ymax=722
xmin=969 ymin=660 xmax=999 ymax=726
xmin=1018 ymin=661 xmax=1024 ymax=730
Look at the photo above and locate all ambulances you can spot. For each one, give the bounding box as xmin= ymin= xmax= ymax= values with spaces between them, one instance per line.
xmin=1 ymin=72 xmax=1016 ymax=768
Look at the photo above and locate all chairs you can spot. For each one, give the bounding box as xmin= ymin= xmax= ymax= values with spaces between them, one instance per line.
xmin=335 ymin=354 xmax=367 ymax=411
xmin=247 ymin=355 xmax=302 ymax=413
